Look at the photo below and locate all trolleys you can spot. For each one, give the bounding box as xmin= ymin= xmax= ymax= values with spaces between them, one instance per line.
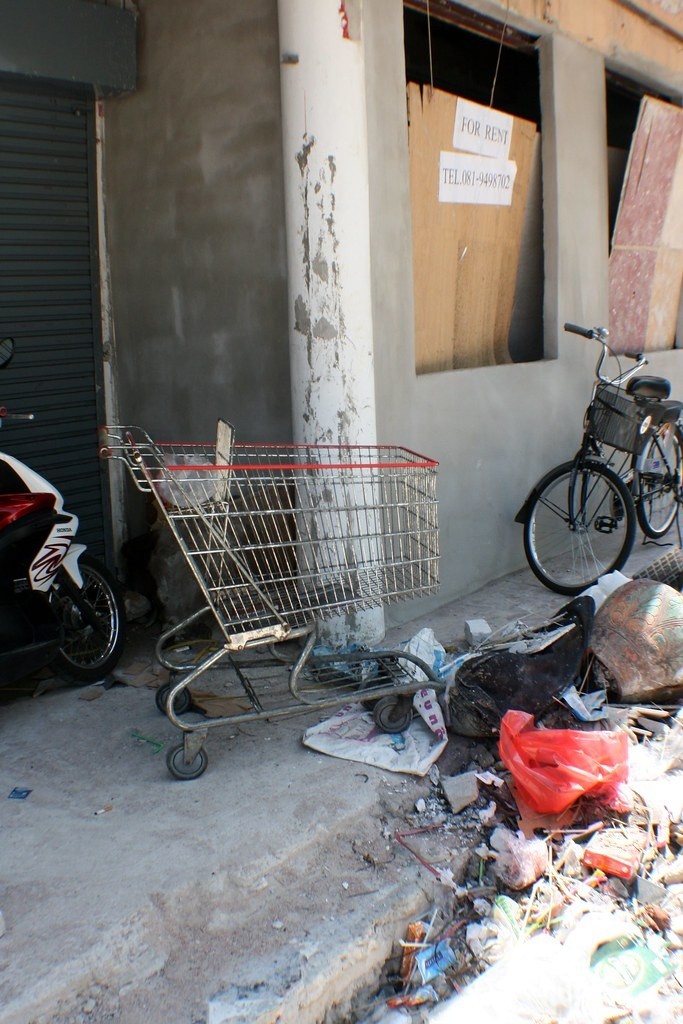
xmin=98 ymin=419 xmax=452 ymax=787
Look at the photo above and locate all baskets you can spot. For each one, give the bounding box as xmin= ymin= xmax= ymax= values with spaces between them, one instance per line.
xmin=587 ymin=383 xmax=665 ymax=455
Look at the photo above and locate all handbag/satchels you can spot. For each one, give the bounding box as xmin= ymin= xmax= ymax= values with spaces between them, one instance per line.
xmin=158 ymin=454 xmax=220 ymax=507
xmin=497 ymin=709 xmax=631 ymax=815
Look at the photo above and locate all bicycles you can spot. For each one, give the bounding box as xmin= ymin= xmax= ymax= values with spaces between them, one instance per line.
xmin=513 ymin=321 xmax=683 ymax=596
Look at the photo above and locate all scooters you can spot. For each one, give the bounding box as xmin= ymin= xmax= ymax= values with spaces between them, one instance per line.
xmin=0 ymin=455 xmax=123 ymax=689
